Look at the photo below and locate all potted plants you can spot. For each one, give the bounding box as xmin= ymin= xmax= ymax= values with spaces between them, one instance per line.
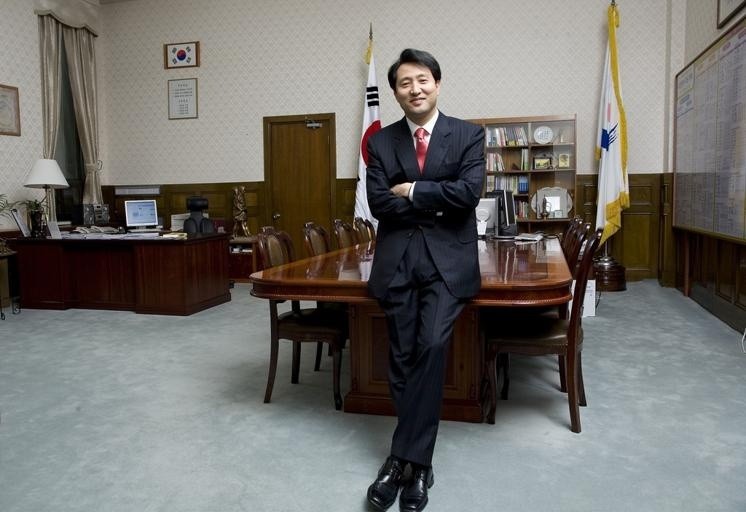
xmin=16 ymin=196 xmax=53 ymax=237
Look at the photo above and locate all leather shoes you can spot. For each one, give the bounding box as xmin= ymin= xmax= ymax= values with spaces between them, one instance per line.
xmin=366 ymin=456 xmax=408 ymax=512
xmin=399 ymin=461 xmax=435 ymax=512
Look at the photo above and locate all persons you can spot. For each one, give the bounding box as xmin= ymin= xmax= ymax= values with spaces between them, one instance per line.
xmin=365 ymin=48 xmax=486 ymax=512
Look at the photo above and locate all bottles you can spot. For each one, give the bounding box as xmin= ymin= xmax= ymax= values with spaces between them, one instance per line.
xmin=541 ymin=197 xmax=548 ymax=220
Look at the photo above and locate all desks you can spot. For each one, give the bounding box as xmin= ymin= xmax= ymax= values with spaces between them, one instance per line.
xmin=230 ymin=233 xmax=261 ymax=284
xmin=6 ymin=224 xmax=236 ymax=319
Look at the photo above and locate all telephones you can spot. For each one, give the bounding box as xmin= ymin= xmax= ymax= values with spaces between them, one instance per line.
xmin=73 ymin=226 xmax=119 ymax=234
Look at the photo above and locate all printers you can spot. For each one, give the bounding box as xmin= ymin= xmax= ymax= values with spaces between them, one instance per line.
xmin=171 ymin=212 xmax=209 ymax=231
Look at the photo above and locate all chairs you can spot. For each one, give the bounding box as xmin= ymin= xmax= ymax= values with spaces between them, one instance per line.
xmin=183 ymin=194 xmax=217 ymax=235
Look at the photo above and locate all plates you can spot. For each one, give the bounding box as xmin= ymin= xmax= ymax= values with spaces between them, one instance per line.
xmin=534 ymin=125 xmax=554 ymax=145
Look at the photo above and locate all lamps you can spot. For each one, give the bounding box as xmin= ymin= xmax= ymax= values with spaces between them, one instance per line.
xmin=27 ymin=156 xmax=70 ymax=235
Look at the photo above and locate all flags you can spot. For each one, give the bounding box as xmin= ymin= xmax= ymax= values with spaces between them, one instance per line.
xmin=350 ymin=32 xmax=383 ymax=236
xmin=592 ymin=0 xmax=631 ymax=252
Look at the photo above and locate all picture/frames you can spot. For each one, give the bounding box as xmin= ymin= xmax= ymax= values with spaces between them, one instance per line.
xmin=167 ymin=77 xmax=201 ymax=121
xmin=713 ymin=0 xmax=746 ymax=33
xmin=162 ymin=39 xmax=203 ymax=70
xmin=0 ymin=82 xmax=23 ymax=139
xmin=536 ymin=188 xmax=569 ymax=219
xmin=532 ymin=155 xmax=552 ymax=171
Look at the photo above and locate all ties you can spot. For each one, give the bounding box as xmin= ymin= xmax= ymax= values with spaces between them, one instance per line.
xmin=414 ymin=128 xmax=429 ymax=175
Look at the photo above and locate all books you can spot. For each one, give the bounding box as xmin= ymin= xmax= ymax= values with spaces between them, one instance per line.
xmin=481 ymin=124 xmax=530 ymax=219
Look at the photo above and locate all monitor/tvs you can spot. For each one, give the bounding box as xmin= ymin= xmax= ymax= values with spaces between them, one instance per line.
xmin=124 ymin=199 xmax=162 ymax=232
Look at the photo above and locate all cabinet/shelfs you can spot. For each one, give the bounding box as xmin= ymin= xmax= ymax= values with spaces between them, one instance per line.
xmin=467 ymin=113 xmax=580 ymax=239
